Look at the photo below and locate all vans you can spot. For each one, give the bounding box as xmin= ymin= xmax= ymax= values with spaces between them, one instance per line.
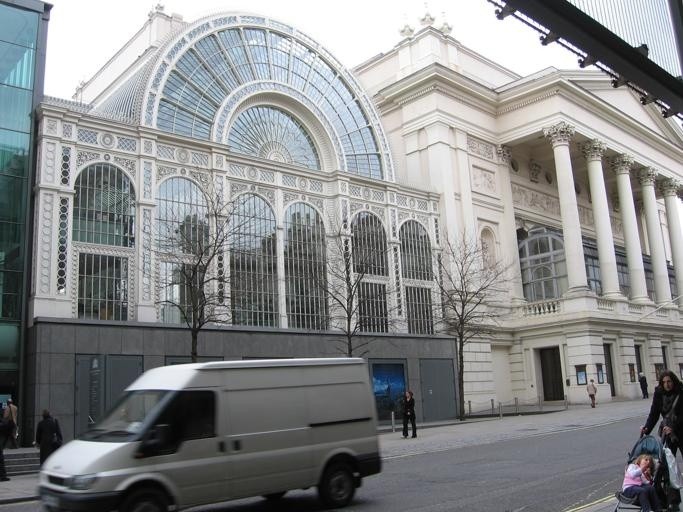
xmin=36 ymin=357 xmax=381 ymax=512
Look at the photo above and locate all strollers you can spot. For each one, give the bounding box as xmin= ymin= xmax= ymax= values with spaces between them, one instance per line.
xmin=614 ymin=426 xmax=682 ymax=512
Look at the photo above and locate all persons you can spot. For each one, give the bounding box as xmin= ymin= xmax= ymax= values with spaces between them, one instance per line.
xmin=587 ymin=379 xmax=597 ymax=408
xmin=3 ymin=398 xmax=19 ymax=448
xmin=638 ymin=371 xmax=649 ymax=399
xmin=621 ymin=453 xmax=663 ymax=512
xmin=0 ymin=418 xmax=16 ymax=481
xmin=640 ymin=370 xmax=683 ymax=512
xmin=400 ymin=390 xmax=418 ymax=440
xmin=32 ymin=409 xmax=63 ymax=467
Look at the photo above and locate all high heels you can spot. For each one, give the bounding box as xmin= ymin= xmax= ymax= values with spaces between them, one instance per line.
xmin=400 ymin=435 xmax=408 ymax=439
xmin=411 ymin=435 xmax=417 ymax=438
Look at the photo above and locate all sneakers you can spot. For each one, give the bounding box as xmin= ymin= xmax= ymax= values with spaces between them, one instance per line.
xmin=655 ymin=508 xmax=668 ymax=512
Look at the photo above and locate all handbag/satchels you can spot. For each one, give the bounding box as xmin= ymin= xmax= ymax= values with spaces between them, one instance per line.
xmin=8 ymin=420 xmax=14 ymax=429
xmin=52 ymin=433 xmax=63 ymax=446
xmin=13 ymin=426 xmax=19 ymax=439
xmin=658 ymin=413 xmax=677 ymax=438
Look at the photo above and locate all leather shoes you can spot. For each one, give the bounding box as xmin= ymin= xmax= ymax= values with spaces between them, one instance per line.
xmin=1 ymin=477 xmax=10 ymax=481
xmin=668 ymin=504 xmax=680 ymax=511
